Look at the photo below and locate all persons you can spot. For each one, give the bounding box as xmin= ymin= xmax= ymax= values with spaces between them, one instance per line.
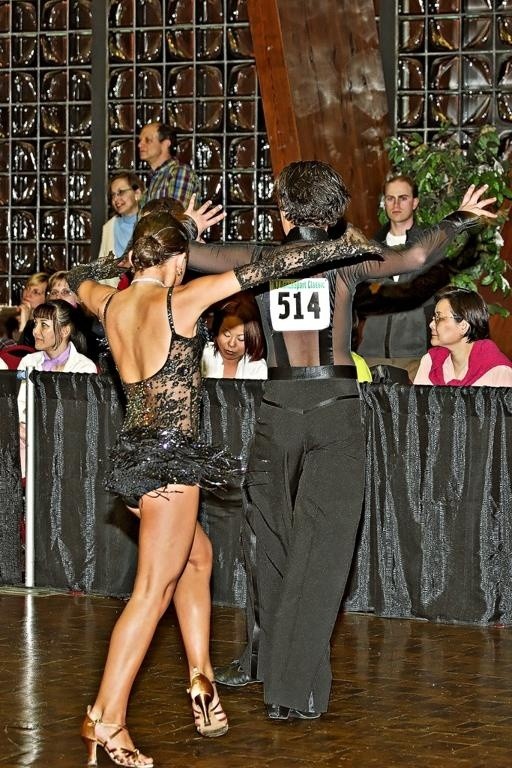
xmin=10 ymin=273 xmax=50 ymax=341
xmin=17 ymin=297 xmax=99 ymax=374
xmin=96 ymin=173 xmax=144 ymax=289
xmin=353 ymin=173 xmax=438 ymax=384
xmin=199 ymin=292 xmax=269 ymax=380
xmin=411 ymin=285 xmax=512 ymax=386
xmin=17 ymin=269 xmax=100 ymax=370
xmin=181 ymin=159 xmax=501 ymax=720
xmin=64 ymin=212 xmax=385 ymax=768
xmin=139 ymin=120 xmax=203 ymax=214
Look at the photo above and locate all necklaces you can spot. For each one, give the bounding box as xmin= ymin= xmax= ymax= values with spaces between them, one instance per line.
xmin=130 ymin=278 xmax=167 ymax=290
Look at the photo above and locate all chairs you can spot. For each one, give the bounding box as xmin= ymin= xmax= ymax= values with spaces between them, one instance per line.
xmin=369 ymin=365 xmax=408 ymax=385
xmin=0 ymin=346 xmax=36 ymax=370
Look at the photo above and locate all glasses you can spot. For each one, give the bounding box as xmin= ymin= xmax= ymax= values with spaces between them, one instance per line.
xmin=432 ymin=313 xmax=462 ymax=325
xmin=109 ymin=188 xmax=133 ymax=196
xmin=47 ymin=288 xmax=71 ymax=297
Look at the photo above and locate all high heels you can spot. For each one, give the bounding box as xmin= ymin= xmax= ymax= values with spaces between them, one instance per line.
xmin=187 ymin=665 xmax=229 ymax=738
xmin=213 ymin=658 xmax=322 ymax=721
xmin=81 ymin=704 xmax=155 ymax=768
